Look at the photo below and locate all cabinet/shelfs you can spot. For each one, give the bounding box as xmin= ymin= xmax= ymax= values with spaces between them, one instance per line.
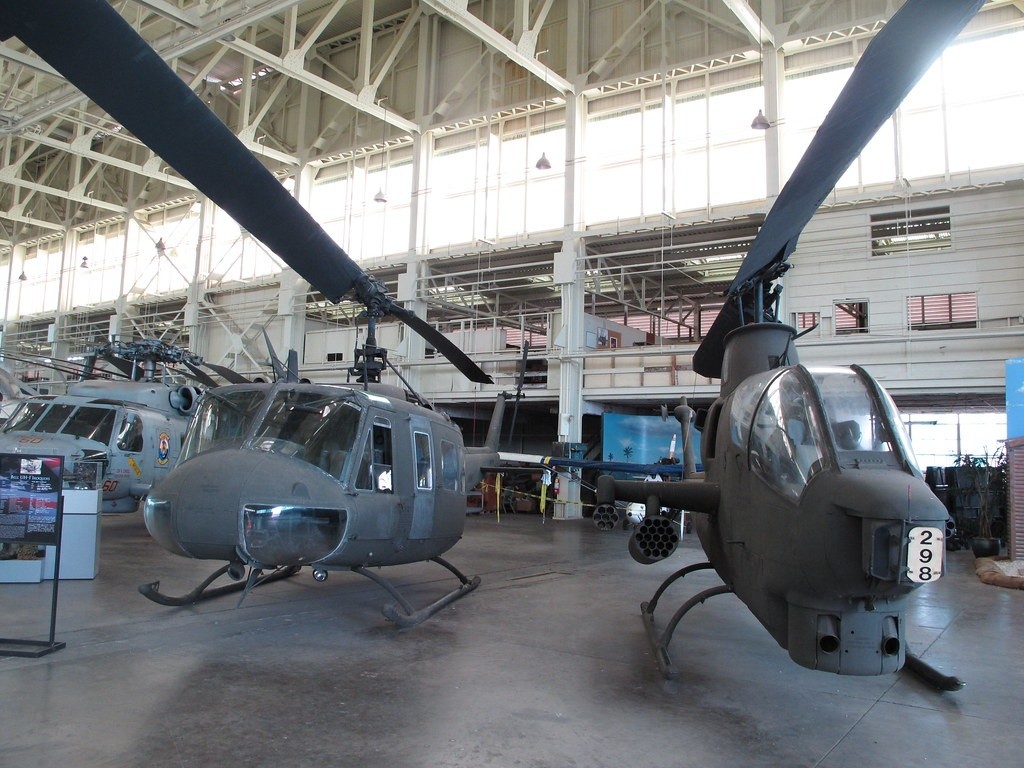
xmin=0 ymin=461 xmax=103 ymax=583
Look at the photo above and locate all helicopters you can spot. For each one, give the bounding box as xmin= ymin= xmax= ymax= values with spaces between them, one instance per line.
xmin=540 ymin=0 xmax=1024 ymax=692
xmin=0 ymin=0 xmax=549 ymax=629
xmin=0 ymin=333 xmax=205 ymax=515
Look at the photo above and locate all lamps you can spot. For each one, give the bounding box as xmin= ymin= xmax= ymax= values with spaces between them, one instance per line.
xmin=81 ymin=191 xmax=92 ymax=268
xmin=751 ymin=0 xmax=771 ymax=130
xmin=18 ymin=210 xmax=29 ymax=281
xmin=536 ymin=51 xmax=551 ymax=171
xmin=374 ymin=96 xmax=387 ymax=202
xmin=155 ymin=168 xmax=170 ymax=250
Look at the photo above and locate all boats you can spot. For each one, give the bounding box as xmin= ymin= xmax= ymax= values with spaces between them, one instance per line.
xmin=658 ymin=434 xmax=680 ymax=465
